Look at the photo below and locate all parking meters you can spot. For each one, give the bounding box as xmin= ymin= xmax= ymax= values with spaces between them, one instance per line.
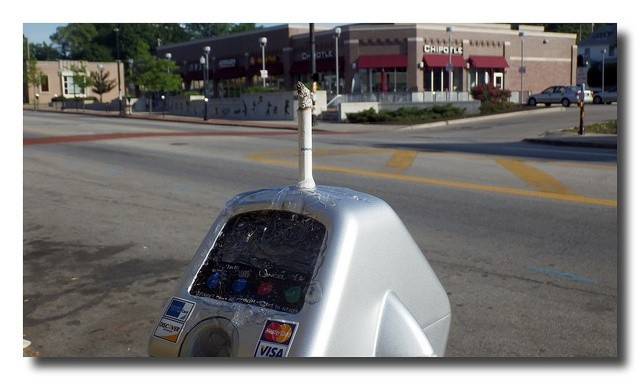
xmin=149 ymin=186 xmax=451 ymax=358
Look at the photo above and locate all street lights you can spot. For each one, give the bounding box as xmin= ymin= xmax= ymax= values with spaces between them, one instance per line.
xmin=444 ymin=26 xmax=453 ymax=100
xmin=166 ymin=53 xmax=171 ymax=74
xmin=97 ymin=64 xmax=105 ymax=98
xmin=259 ymin=36 xmax=267 ymax=88
xmin=601 ymin=49 xmax=606 ymax=93
xmin=200 ymin=56 xmax=207 ymax=120
xmin=336 ymin=28 xmax=341 ymax=94
xmin=204 ymin=44 xmax=211 ymax=81
xmin=113 ymin=28 xmax=123 ymax=115
xmin=519 ymin=31 xmax=524 ymax=107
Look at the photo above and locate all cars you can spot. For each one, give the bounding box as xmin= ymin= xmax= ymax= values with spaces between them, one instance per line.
xmin=526 ymin=85 xmax=594 ymax=106
xmin=594 ymin=88 xmax=617 ymax=104
